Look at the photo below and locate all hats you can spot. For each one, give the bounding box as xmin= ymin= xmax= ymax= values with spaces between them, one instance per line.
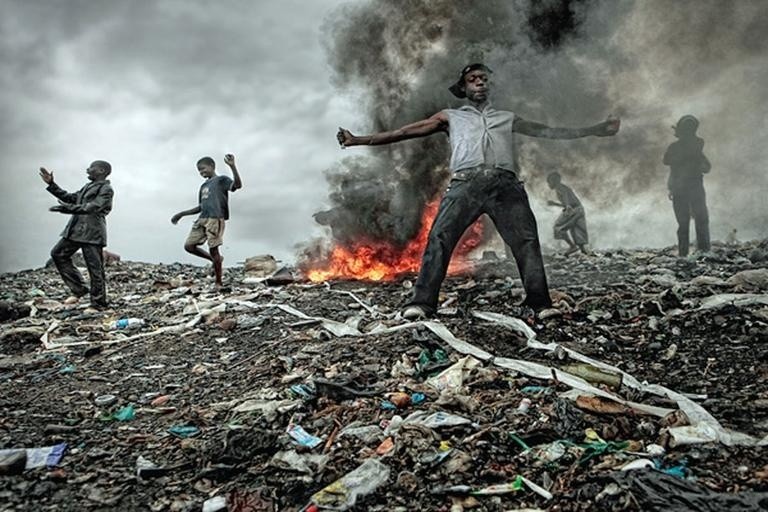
xmin=672 ymin=115 xmax=699 ymax=133
xmin=448 ymin=64 xmax=492 ymax=100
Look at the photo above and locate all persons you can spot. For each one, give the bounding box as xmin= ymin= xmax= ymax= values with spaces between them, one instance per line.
xmin=726 ymin=229 xmax=737 ymax=248
xmin=39 ymin=161 xmax=114 ymax=314
xmin=663 ymin=116 xmax=719 ymax=261
xmin=336 ymin=63 xmax=620 ymax=319
xmin=544 ymin=171 xmax=589 ymax=255
xmin=171 ymin=153 xmax=242 ymax=289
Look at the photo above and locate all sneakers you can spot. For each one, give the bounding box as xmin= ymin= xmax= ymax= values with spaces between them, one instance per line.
xmin=210 ymin=255 xmax=225 ymax=289
xmin=534 ymin=308 xmax=563 ymax=318
xmin=64 ymin=290 xmax=105 ymax=312
xmin=564 ymin=246 xmax=578 ymax=255
xmin=403 ymin=306 xmax=426 ymax=318
